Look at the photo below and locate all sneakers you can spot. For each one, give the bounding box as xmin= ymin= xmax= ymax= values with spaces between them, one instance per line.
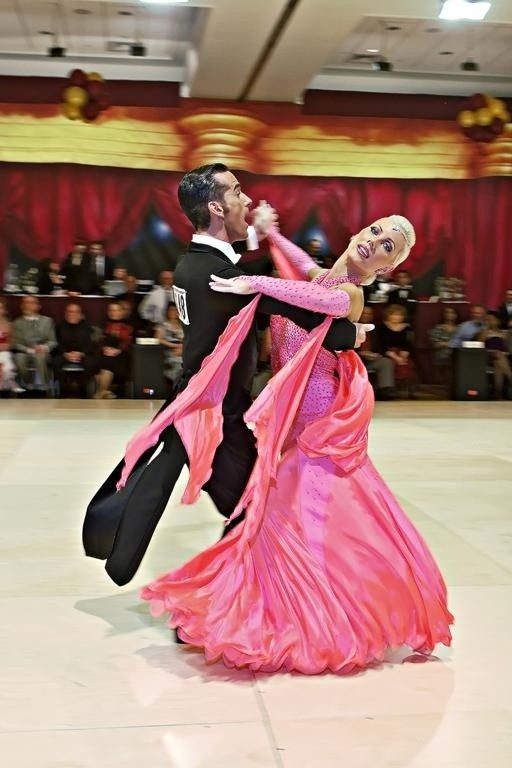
xmin=379 ymin=384 xmax=445 ymax=402
xmin=7 ymin=380 xmax=121 ymax=401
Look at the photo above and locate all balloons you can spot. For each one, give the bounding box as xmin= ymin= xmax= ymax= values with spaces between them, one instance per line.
xmin=455 ymin=94 xmax=510 ymax=144
xmin=62 ymin=68 xmax=113 ymax=123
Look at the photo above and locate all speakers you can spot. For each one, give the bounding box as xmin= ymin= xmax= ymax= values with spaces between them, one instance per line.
xmin=53 ymin=362 xmax=87 ymax=399
xmin=448 ymin=339 xmax=490 ymax=401
xmin=130 ymin=342 xmax=171 ymax=400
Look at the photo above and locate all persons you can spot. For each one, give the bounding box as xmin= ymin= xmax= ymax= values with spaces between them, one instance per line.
xmin=171 ymin=160 xmax=381 ymax=649
xmin=0 ymin=239 xmax=188 ymax=399
xmin=425 ymin=286 xmax=510 ymax=401
xmin=207 ymin=196 xmax=417 ymax=675
xmin=307 ymin=239 xmax=335 ymax=269
xmin=356 ymin=269 xmax=427 ymax=401
